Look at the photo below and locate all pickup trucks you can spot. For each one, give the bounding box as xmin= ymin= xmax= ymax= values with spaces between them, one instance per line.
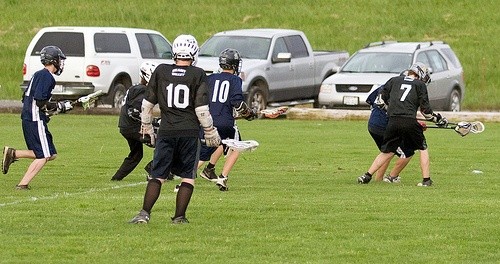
xmin=194 ymin=28 xmax=352 ymax=121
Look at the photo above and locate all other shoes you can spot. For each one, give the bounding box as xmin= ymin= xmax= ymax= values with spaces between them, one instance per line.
xmin=147 ymin=172 xmax=180 ymax=182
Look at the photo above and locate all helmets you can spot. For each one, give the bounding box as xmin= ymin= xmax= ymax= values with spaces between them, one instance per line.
xmin=400 ymin=62 xmax=432 ymax=87
xmin=40 ymin=46 xmax=67 ymax=76
xmin=219 ymin=48 xmax=243 ymax=76
xmin=139 ymin=62 xmax=158 ymax=83
xmin=171 ymin=34 xmax=200 ymax=67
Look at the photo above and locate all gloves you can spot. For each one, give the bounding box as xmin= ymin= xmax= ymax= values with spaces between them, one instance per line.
xmin=434 ymin=113 xmax=449 ymax=128
xmin=418 ymin=121 xmax=427 ymax=131
xmin=139 ymin=123 xmax=157 ymax=149
xmin=56 ymin=100 xmax=74 ymax=114
xmin=242 ymin=108 xmax=258 ymax=121
xmin=204 ymin=127 xmax=221 ymax=147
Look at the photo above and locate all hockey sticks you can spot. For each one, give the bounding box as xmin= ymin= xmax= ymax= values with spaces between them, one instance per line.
xmin=426 ymin=122 xmax=471 ymax=137
xmin=45 ymin=90 xmax=102 ymax=118
xmin=416 ymin=117 xmax=485 ymax=134
xmin=234 ymin=106 xmax=288 ymax=119
xmin=140 ymin=136 xmax=259 ymax=152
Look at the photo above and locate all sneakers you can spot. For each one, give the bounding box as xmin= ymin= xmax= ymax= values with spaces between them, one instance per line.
xmin=359 ymin=173 xmax=373 ymax=183
xmin=1 ymin=146 xmax=19 ymax=175
xmin=14 ymin=184 xmax=32 ymax=191
xmin=170 ymin=213 xmax=190 ymax=226
xmin=216 ymin=176 xmax=228 ymax=191
xmin=128 ymin=209 xmax=150 ymax=224
xmin=173 ymin=184 xmax=180 ymax=191
xmin=199 ymin=164 xmax=218 ymax=182
xmin=422 ymin=180 xmax=434 ymax=186
xmin=383 ymin=173 xmax=402 ymax=183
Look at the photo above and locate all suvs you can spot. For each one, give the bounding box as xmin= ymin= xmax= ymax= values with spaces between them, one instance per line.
xmin=315 ymin=41 xmax=465 ymax=117
xmin=19 ymin=26 xmax=177 ymax=104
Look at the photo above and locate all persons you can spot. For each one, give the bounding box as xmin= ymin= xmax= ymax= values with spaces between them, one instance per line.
xmin=126 ymin=34 xmax=221 ymax=225
xmin=2 ymin=45 xmax=75 ymax=190
xmin=365 ymin=69 xmax=427 ymax=184
xmin=357 ymin=61 xmax=449 ymax=187
xmin=110 ymin=47 xmax=258 ymax=191
